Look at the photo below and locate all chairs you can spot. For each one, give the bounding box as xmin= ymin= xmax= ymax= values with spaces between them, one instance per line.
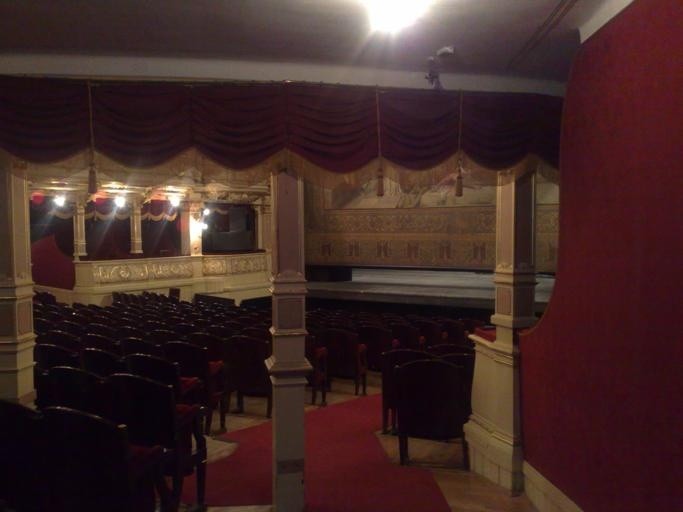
xmin=305 ymin=299 xmax=485 ymax=467
xmin=2 ymin=289 xmax=272 ymax=511
